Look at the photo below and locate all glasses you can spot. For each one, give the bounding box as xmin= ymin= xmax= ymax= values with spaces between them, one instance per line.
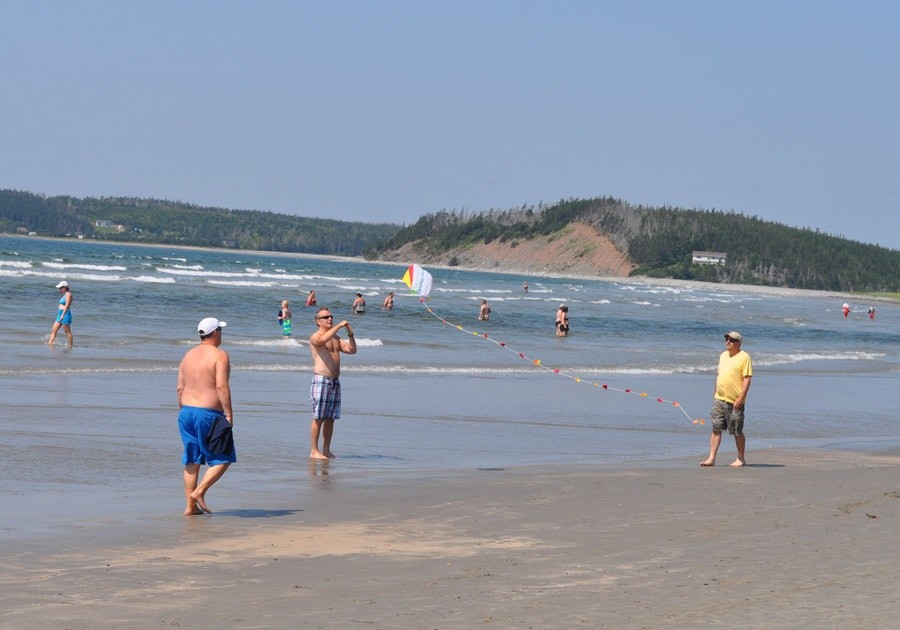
xmin=319 ymin=315 xmax=333 ymax=319
xmin=216 ymin=328 xmax=221 ymax=331
xmin=726 ymin=337 xmax=738 ymax=342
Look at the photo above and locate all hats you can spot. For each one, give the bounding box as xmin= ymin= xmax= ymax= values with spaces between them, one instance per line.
xmin=724 ymin=331 xmax=742 ymax=342
xmin=56 ymin=281 xmax=68 ymax=288
xmin=198 ymin=318 xmax=227 ymax=336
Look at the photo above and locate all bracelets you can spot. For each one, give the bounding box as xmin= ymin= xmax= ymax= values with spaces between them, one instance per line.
xmin=348 ymin=333 xmax=354 ymax=337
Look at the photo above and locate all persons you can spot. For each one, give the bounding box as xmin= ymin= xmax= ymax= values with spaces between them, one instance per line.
xmin=383 ymin=292 xmax=394 ymax=310
xmin=478 ymin=299 xmax=491 ymax=320
xmin=869 ymin=306 xmax=877 ymax=320
xmin=556 ymin=305 xmax=569 ymax=337
xmin=523 ymin=281 xmax=529 ymax=293
xmin=308 ymin=289 xmax=317 ymax=306
xmin=700 ymin=331 xmax=752 ymax=467
xmin=843 ymin=303 xmax=850 ymax=320
xmin=309 ymin=306 xmax=357 ymax=459
xmin=49 ymin=281 xmax=73 ymax=346
xmin=278 ymin=300 xmax=293 ymax=339
xmin=176 ymin=318 xmax=237 ymax=516
xmin=353 ymin=293 xmax=366 ymax=314
